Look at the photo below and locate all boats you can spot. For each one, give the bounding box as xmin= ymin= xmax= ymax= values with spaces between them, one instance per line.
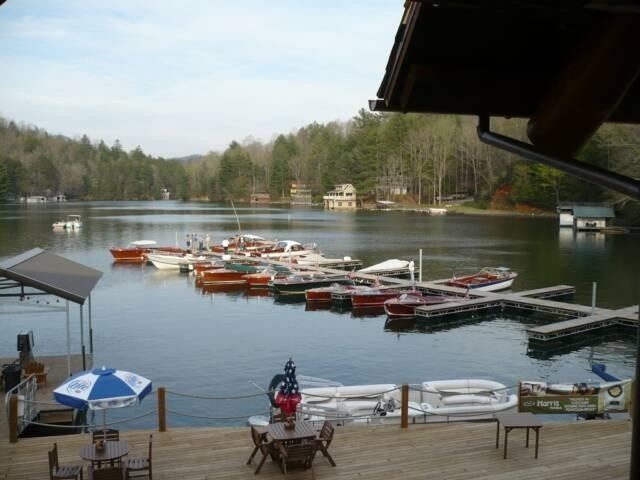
xmin=244 ymin=372 xmax=520 ymax=427
xmin=520 ymin=376 xmax=602 ymax=396
xmin=381 ymin=312 xmax=500 ymax=334
xmin=52 ymin=214 xmax=82 ymax=229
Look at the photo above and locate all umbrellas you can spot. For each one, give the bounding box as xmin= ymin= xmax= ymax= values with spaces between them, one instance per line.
xmin=52 ymin=365 xmax=153 ymax=442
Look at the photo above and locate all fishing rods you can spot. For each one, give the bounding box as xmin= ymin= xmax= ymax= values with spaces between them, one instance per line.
xmin=230 ymin=199 xmax=242 ymax=237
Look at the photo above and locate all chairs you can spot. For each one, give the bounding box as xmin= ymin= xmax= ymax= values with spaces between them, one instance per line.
xmin=87 ymin=460 xmax=127 ymax=480
xmin=125 ymin=434 xmax=152 ymax=480
xmin=246 ymin=420 xmax=336 ymax=480
xmin=48 ymin=442 xmax=83 ymax=480
xmin=93 ymin=429 xmax=119 ymax=468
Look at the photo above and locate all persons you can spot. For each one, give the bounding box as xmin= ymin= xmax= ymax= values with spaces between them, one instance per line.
xmin=183 ymin=233 xmax=212 ymax=253
xmin=220 ymin=234 xmax=246 ymax=255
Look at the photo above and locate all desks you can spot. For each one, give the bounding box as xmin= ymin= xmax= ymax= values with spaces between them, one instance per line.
xmin=494 ymin=413 xmax=544 ymax=460
xmin=22 ymin=367 xmax=50 ymax=389
